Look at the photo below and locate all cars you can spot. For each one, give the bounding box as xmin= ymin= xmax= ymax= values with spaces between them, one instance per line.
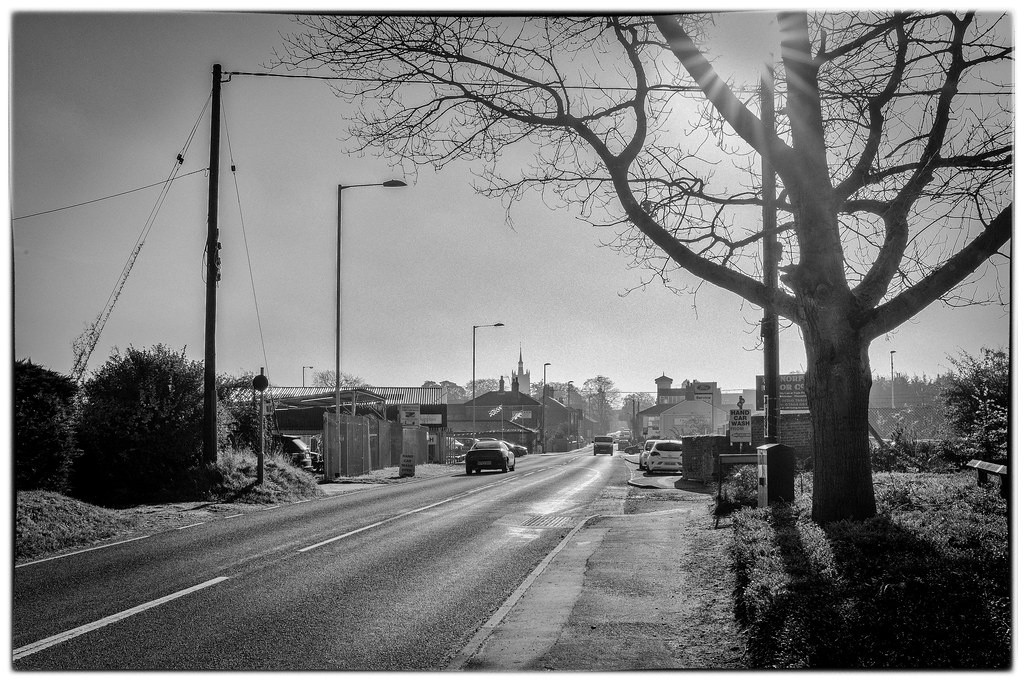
xmin=447 ymin=434 xmax=530 ymax=458
xmin=465 ymin=440 xmax=516 ymax=475
xmin=624 ymin=441 xmax=645 ymax=454
xmin=638 ymin=439 xmax=663 ymax=470
xmin=645 ymin=440 xmax=683 ymax=474
xmin=608 ymin=427 xmax=632 ymax=451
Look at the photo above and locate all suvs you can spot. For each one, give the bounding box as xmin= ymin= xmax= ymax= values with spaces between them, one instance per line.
xmin=591 ymin=435 xmax=616 ymax=456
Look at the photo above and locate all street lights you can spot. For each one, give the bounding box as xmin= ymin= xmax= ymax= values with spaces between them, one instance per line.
xmin=333 ymin=179 xmax=411 ymax=479
xmin=889 ymin=349 xmax=898 ymax=409
xmin=567 ymin=381 xmax=575 ymax=452
xmin=542 ymin=362 xmax=552 ymax=454
xmin=472 ymin=322 xmax=505 ymax=446
xmin=588 ymin=396 xmax=593 ymax=445
xmin=302 ymin=365 xmax=315 ymax=394
